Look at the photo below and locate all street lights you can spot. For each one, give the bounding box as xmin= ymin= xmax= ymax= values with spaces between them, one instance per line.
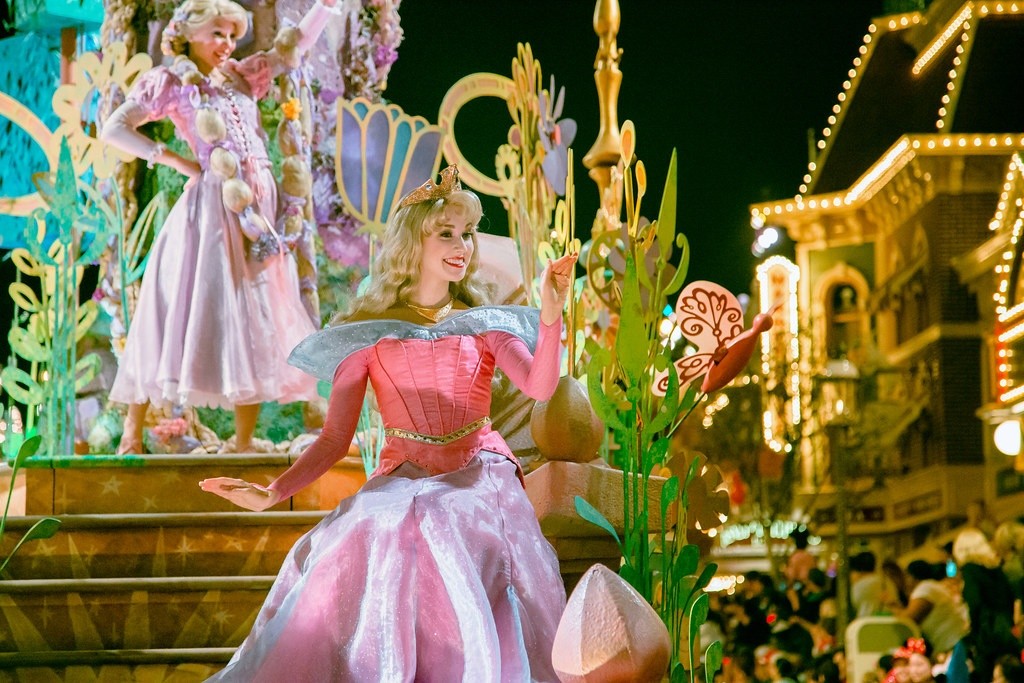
xmin=808 ymin=356 xmax=860 ymax=649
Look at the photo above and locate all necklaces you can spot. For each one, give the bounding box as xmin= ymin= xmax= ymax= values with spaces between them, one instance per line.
xmin=402 ymin=293 xmax=455 ymax=324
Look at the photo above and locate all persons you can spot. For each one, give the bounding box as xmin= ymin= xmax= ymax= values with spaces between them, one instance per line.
xmin=199 ymin=166 xmax=580 ymax=683
xmin=101 ymin=0 xmax=336 ymax=455
xmin=699 ymin=499 xmax=1024 ymax=683
